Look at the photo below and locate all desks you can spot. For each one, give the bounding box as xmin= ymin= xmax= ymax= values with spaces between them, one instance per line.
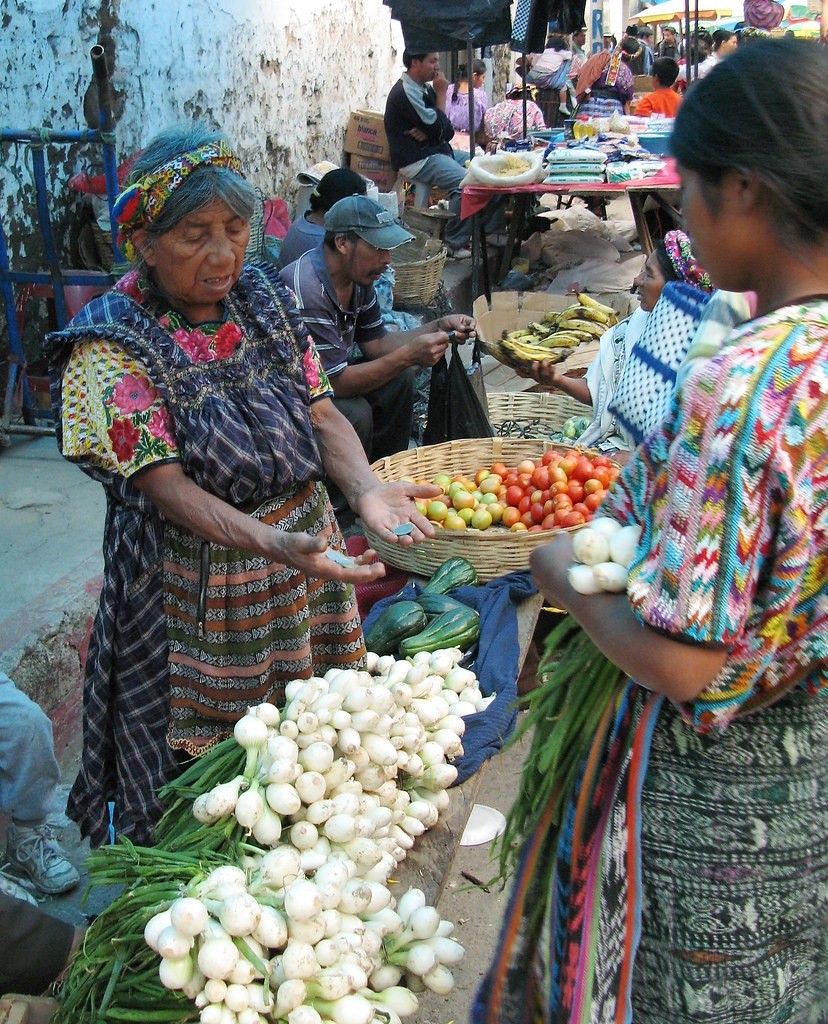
xmin=460 ymin=122 xmax=689 ymax=293
xmin=386 ymin=593 xmax=547 ymax=916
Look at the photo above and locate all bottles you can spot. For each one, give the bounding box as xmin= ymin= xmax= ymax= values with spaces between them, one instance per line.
xmin=573 ymin=113 xmax=603 ymax=140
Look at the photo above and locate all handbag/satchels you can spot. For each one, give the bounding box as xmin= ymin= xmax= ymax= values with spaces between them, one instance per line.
xmin=422 ymin=328 xmax=494 ymax=446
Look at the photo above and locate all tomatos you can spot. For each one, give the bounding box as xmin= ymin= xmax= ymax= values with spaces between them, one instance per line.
xmin=399 ymin=450 xmax=620 ymax=534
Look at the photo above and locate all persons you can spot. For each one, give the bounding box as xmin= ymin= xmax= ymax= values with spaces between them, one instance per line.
xmin=280 ymin=168 xmax=477 ymax=512
xmin=41 ymin=127 xmax=443 ymax=849
xmin=0 ymin=670 xmax=80 ymax=893
xmin=382 ymin=0 xmax=828 ymax=258
xmin=514 ymin=231 xmax=718 ymax=452
xmin=473 ymin=38 xmax=828 ymax=1024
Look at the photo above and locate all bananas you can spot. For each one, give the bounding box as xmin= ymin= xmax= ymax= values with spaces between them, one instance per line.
xmin=497 ymin=290 xmax=621 ymax=368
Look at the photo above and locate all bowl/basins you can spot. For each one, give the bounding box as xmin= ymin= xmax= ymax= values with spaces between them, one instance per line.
xmin=637 ymin=131 xmax=673 ymax=154
xmin=528 ymin=131 xmax=563 ymax=140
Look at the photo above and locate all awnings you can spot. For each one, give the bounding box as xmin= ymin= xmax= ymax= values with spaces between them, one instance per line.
xmin=627 ymin=0 xmax=745 ymax=59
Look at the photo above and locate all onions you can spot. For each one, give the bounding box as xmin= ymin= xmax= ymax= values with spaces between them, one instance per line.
xmin=567 ymin=517 xmax=643 ymax=594
xmin=143 ymin=645 xmax=498 ymax=1024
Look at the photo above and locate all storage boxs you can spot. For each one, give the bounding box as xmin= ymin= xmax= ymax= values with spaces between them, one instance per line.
xmin=342 ymin=109 xmax=401 ymax=190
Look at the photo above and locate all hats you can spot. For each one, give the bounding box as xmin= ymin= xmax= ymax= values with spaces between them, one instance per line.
xmin=309 ymin=168 xmax=367 ymax=208
xmin=322 ymin=195 xmax=417 ymax=251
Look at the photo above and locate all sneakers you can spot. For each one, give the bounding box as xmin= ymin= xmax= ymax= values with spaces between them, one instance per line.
xmin=1 ymin=877 xmax=39 ymax=907
xmin=5 ymin=826 xmax=81 ymax=894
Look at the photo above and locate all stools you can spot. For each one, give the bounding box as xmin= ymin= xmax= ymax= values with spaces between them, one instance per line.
xmin=398 ymin=175 xmax=430 ymax=218
xmin=8 ymin=271 xmax=115 ymax=395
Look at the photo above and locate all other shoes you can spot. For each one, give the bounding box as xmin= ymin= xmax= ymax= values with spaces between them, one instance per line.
xmin=487 ymin=234 xmax=525 ymax=247
xmin=448 ymin=247 xmax=471 ymax=258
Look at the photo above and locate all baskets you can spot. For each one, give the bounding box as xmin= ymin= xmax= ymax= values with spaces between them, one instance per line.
xmin=488 ymin=392 xmax=594 ymax=438
xmin=388 ymin=233 xmax=444 ymax=310
xmin=365 ymin=438 xmax=627 ymax=584
